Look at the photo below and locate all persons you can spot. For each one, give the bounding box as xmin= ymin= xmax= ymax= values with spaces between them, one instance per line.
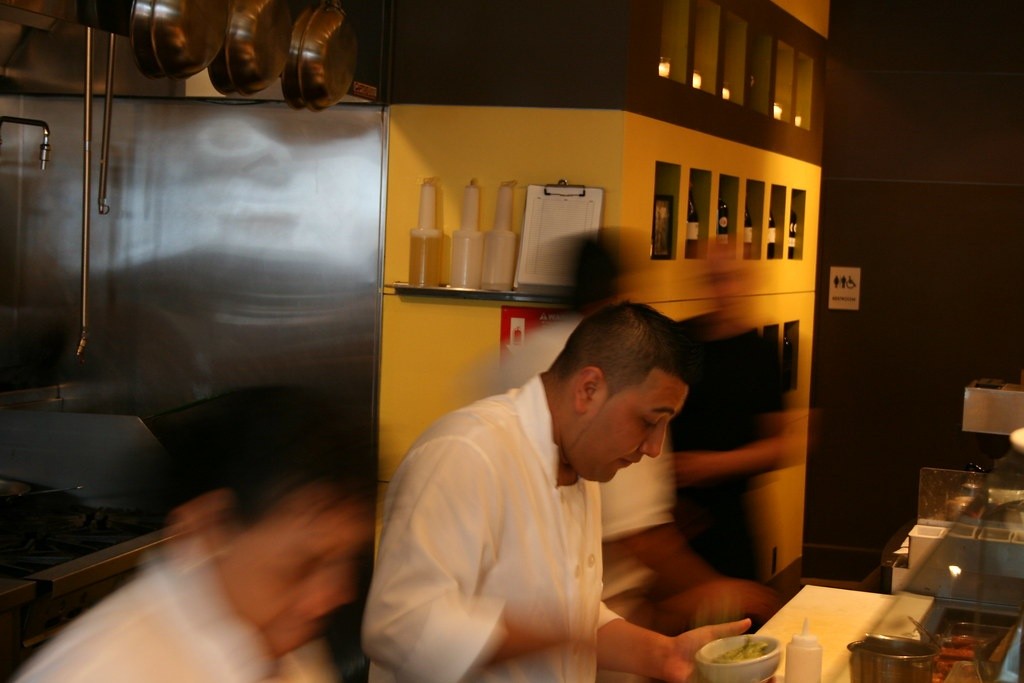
xmin=359 ymin=304 xmax=775 ymax=683
xmin=498 ymin=227 xmax=818 ymax=636
xmin=8 ymin=376 xmax=375 ymax=683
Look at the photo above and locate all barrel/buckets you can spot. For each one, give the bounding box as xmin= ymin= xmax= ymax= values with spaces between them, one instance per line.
xmin=846 ymin=633 xmax=941 ymax=683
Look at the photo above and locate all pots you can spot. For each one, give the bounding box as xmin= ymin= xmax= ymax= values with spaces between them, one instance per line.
xmin=128 ymin=0 xmax=358 ymax=113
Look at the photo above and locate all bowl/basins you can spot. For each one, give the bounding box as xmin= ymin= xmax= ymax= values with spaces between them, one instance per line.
xmin=695 ymin=634 xmax=781 ymax=683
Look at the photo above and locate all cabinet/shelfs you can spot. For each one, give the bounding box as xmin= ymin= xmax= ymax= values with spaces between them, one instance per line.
xmin=377 ymin=0 xmax=831 ymax=578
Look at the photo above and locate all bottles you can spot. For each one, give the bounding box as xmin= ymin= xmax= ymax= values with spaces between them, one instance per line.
xmin=788 ymin=209 xmax=797 ymax=259
xmin=744 ymin=202 xmax=753 ymax=261
xmin=684 ymin=181 xmax=699 ymax=260
xmin=786 ymin=617 xmax=822 ymax=683
xmin=782 ymin=332 xmax=792 ymax=393
xmin=767 ymin=210 xmax=776 ymax=259
xmin=718 ymin=193 xmax=729 ymax=252
xmin=409 ymin=181 xmax=517 ymax=294
